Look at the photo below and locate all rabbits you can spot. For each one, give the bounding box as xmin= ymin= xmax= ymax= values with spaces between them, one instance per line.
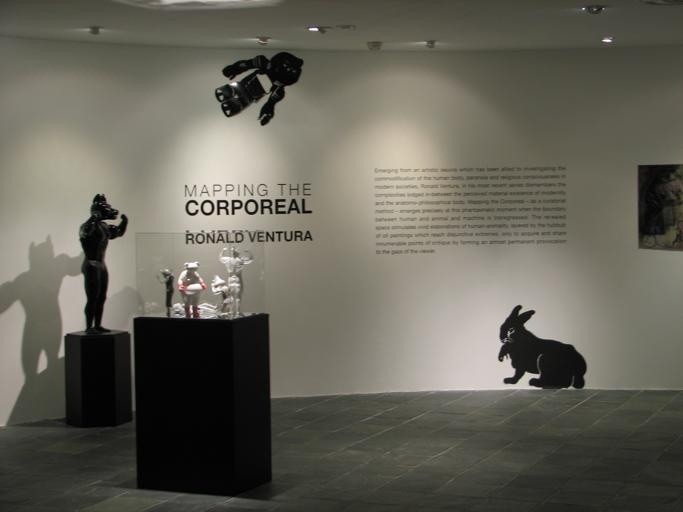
xmin=498 ymin=305 xmax=587 ymax=390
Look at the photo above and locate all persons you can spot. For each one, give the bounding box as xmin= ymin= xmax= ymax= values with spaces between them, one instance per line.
xmin=79 ymin=194 xmax=128 ymax=335
